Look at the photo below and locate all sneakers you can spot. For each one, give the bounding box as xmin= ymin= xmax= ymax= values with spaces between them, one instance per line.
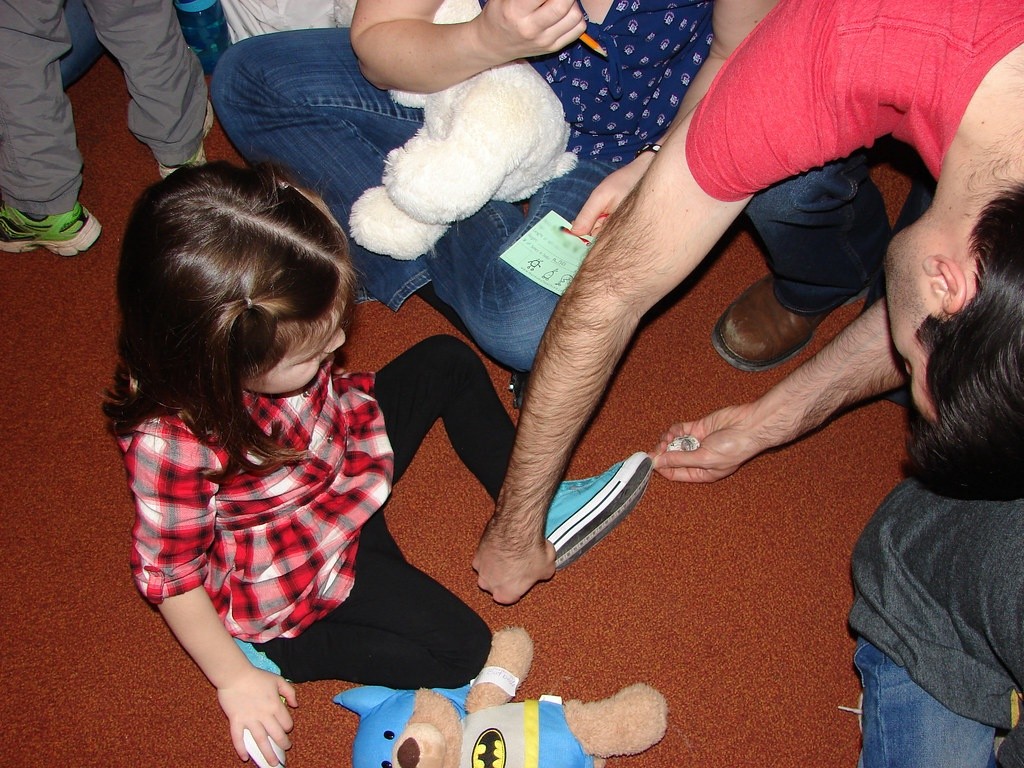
xmin=157 ymin=99 xmax=213 ymax=180
xmin=230 ymin=624 xmax=294 ymax=768
xmin=543 ymin=452 xmax=655 ymax=573
xmin=0 ymin=199 xmax=103 ymax=257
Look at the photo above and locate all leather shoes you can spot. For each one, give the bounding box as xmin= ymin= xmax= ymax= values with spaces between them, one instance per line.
xmin=711 ymin=276 xmax=875 ymax=374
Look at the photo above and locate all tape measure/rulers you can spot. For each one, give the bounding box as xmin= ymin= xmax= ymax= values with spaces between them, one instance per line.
xmin=553 ymin=434 xmax=700 ymax=566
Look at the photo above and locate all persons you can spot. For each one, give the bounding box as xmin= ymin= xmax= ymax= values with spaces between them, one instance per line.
xmin=0 ymin=0 xmax=215 ymax=256
xmin=849 ymin=480 xmax=1024 ymax=768
xmin=102 ymin=158 xmax=653 ymax=768
xmin=209 ymin=0 xmax=778 ymax=372
xmin=472 ymin=0 xmax=1024 ymax=606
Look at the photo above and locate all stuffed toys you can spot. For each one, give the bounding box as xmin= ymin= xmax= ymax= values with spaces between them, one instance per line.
xmin=350 ymin=0 xmax=578 ymax=259
xmin=331 ymin=629 xmax=668 ymax=768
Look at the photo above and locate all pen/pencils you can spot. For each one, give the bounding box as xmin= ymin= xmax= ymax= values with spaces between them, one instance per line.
xmin=580 ymin=32 xmax=608 ymax=57
